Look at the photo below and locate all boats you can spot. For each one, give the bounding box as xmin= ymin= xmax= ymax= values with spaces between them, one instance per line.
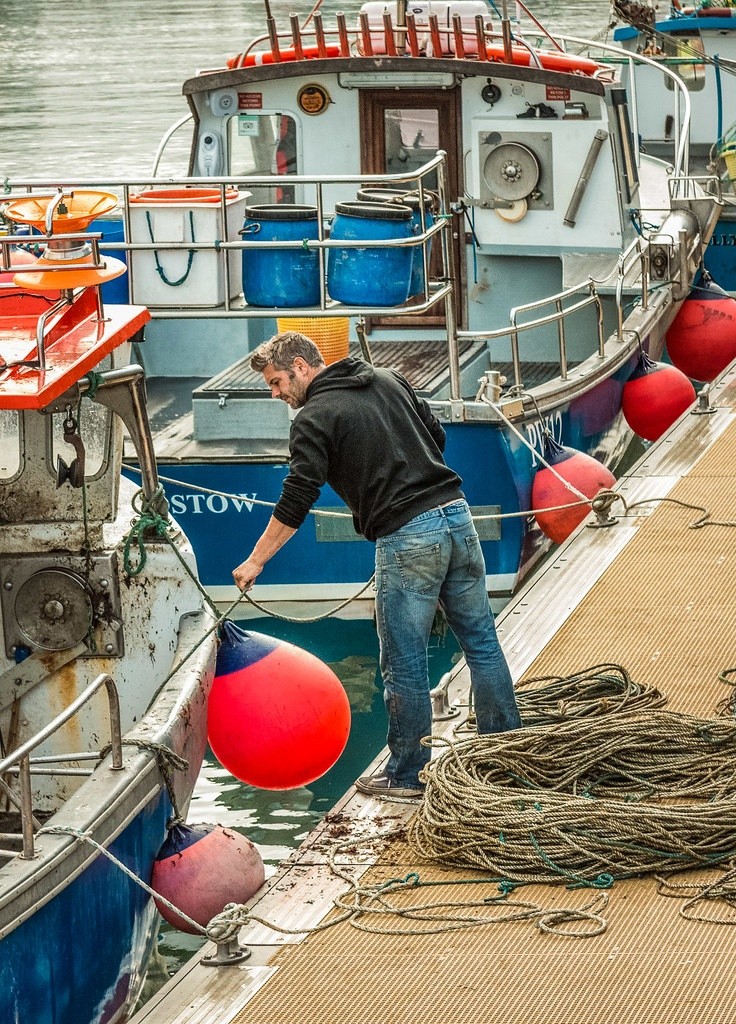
xmin=0 ymin=0 xmax=735 ymax=1024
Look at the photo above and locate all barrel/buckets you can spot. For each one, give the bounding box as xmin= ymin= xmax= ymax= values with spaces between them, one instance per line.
xmin=354 ymin=187 xmax=434 ymax=296
xmin=326 ymin=202 xmax=419 ymax=306
xmin=238 ymin=203 xmax=326 ymax=307
xmin=86 ymin=221 xmax=127 ymax=303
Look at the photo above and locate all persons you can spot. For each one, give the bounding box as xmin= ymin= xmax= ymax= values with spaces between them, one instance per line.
xmin=232 ymin=331 xmax=521 ymax=796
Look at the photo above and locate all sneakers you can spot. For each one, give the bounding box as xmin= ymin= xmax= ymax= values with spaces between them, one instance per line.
xmin=356 ymin=772 xmax=425 ymax=797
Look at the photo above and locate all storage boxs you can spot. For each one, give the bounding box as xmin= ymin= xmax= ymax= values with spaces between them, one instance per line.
xmin=115 ymin=190 xmax=253 ymax=309
